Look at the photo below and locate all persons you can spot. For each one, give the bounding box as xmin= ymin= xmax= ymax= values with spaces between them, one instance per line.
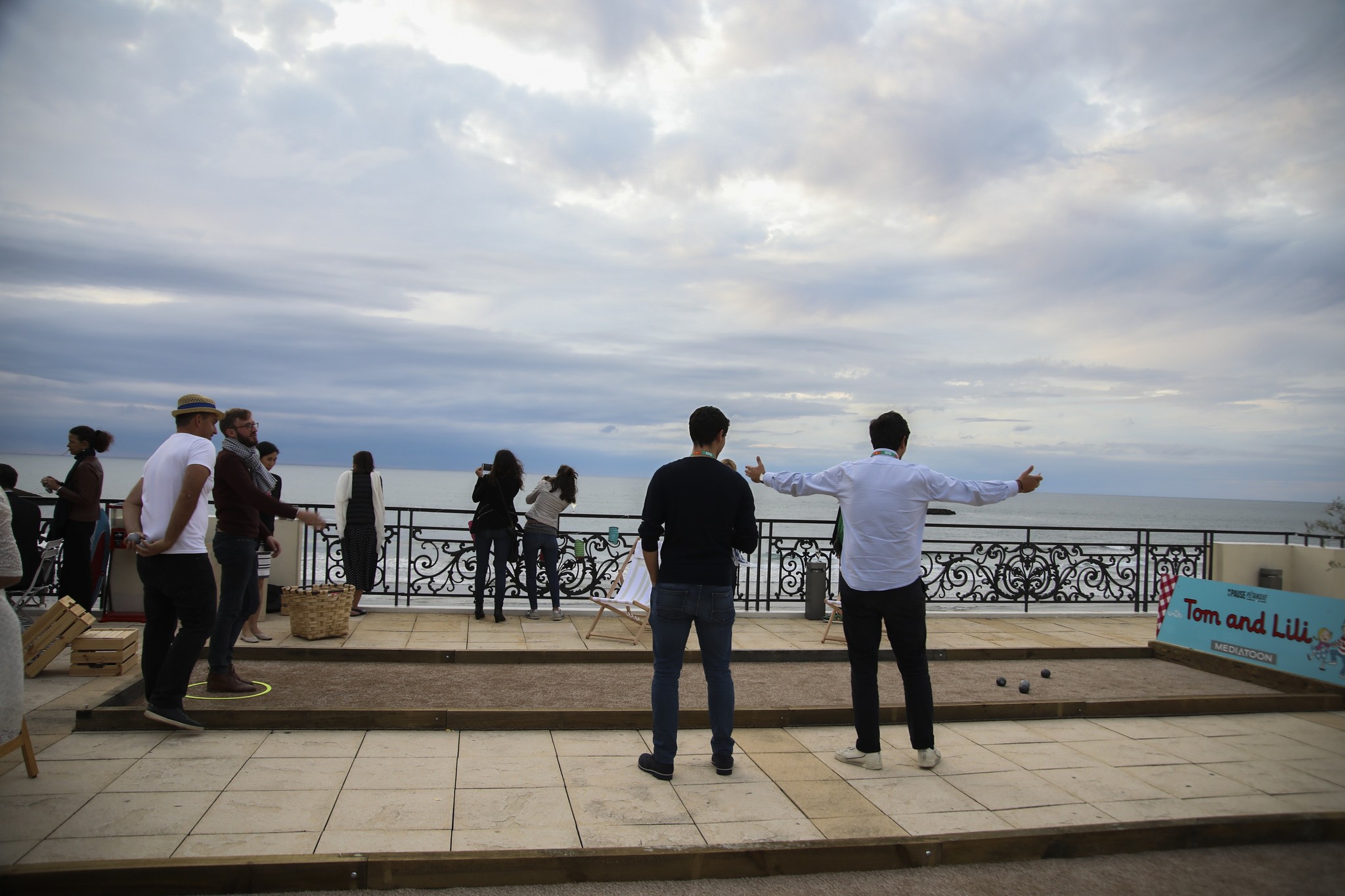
xmin=0 ymin=463 xmax=45 ymax=595
xmin=206 ymin=408 xmax=327 ymax=693
xmin=241 ymin=441 xmax=282 ymax=643
xmin=638 ymin=406 xmax=759 ymax=781
xmin=522 ymin=464 xmax=579 ymax=621
xmin=744 ymin=410 xmax=1044 ymax=771
xmin=40 ymin=425 xmax=115 ymax=614
xmin=122 ymin=394 xmax=226 ymax=731
xmin=0 ymin=485 xmax=23 ymax=589
xmin=472 ymin=449 xmax=527 ymax=623
xmin=334 ymin=450 xmax=386 ymax=617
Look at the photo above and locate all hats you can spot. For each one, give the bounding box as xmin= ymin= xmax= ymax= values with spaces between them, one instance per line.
xmin=171 ymin=394 xmax=226 ymax=421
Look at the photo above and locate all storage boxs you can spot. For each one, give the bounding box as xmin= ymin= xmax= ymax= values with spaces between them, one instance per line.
xmin=69 ymin=628 xmax=139 ymax=677
xmin=21 ymin=595 xmax=97 ymax=678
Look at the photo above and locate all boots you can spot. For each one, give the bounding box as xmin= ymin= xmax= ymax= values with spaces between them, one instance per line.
xmin=474 ymin=599 xmax=485 ymax=620
xmin=494 ymin=596 xmax=506 ymax=623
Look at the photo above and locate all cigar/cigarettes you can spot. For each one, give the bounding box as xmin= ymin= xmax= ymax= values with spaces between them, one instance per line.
xmin=61 ymin=449 xmax=70 ymax=456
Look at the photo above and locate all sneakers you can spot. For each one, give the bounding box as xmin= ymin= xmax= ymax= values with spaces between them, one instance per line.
xmin=143 ymin=700 xmax=205 ymax=732
xmin=552 ymin=608 xmax=565 ymax=621
xmin=834 ymin=747 xmax=883 ymax=770
xmin=524 ymin=609 xmax=540 ymax=619
xmin=822 ymin=612 xmax=843 ymax=624
xmin=917 ymin=747 xmax=941 ymax=767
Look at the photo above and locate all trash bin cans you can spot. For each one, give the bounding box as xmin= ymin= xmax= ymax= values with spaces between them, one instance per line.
xmin=806 ymin=561 xmax=826 ymax=621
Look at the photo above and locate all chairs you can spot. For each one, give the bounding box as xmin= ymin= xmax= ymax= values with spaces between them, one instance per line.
xmin=821 ymin=591 xmax=887 ymax=643
xmin=5 ymin=538 xmax=62 ymax=633
xmin=585 ymin=537 xmax=664 ymax=645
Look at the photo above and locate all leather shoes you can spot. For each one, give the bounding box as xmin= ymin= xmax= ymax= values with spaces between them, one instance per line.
xmin=240 ymin=633 xmax=259 ymax=643
xmin=207 ymin=669 xmax=257 ymax=693
xmin=253 ymin=633 xmax=272 ymax=640
xmin=711 ymin=754 xmax=735 ymax=776
xmin=206 ymin=664 xmax=254 ymax=686
xmin=638 ymin=753 xmax=674 ymax=781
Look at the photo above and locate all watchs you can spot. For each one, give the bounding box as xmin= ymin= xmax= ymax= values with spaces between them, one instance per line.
xmin=759 ymin=472 xmax=766 ymax=484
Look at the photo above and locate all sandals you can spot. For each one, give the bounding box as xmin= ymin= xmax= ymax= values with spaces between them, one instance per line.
xmin=349 ymin=609 xmax=367 ymax=617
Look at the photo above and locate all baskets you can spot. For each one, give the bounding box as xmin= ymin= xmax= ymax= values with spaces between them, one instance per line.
xmin=281 ymin=582 xmax=356 ymax=640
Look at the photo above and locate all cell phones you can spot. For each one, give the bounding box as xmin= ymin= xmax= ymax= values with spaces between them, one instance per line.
xmin=544 ymin=476 xmax=550 ymax=480
xmin=482 ymin=463 xmax=492 ymax=471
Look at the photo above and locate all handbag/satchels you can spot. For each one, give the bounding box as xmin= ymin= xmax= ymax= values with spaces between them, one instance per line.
xmin=507 ymin=524 xmax=519 ymax=562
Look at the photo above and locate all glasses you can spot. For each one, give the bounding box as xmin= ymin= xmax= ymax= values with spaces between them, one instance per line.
xmin=229 ymin=422 xmax=259 ymax=431
